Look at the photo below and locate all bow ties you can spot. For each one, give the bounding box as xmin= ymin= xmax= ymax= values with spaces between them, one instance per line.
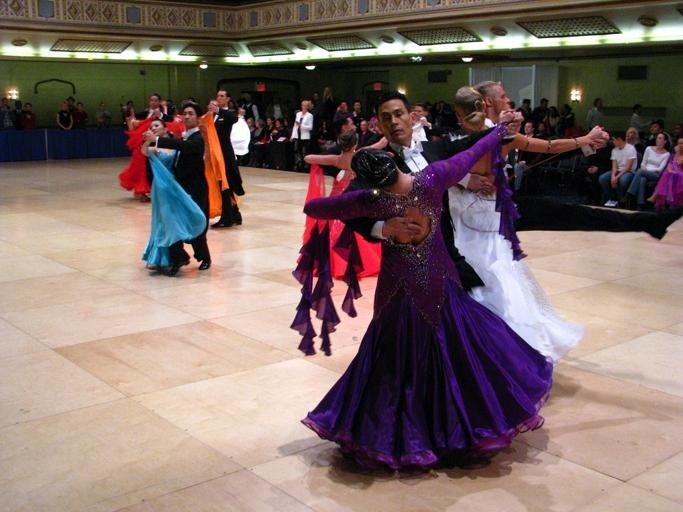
xmin=182 ymin=132 xmax=189 ymax=138
xmin=403 ymin=140 xmax=424 ymax=160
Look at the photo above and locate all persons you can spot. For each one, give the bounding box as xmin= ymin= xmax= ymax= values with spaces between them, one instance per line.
xmin=296 ymin=128 xmax=389 ymax=283
xmin=290 ymin=110 xmax=556 ymax=476
xmin=49 ymin=97 xmax=88 ymax=130
xmin=140 ymin=119 xmax=207 ymax=271
xmin=120 ymin=81 xmax=683 ymax=227
xmin=442 ymin=85 xmax=605 ymax=368
xmin=340 ymin=90 xmax=524 ymax=295
xmin=145 ymin=101 xmax=212 ymax=274
xmin=0 ymin=97 xmax=38 ymax=131
xmin=94 ymin=102 xmax=111 ymax=128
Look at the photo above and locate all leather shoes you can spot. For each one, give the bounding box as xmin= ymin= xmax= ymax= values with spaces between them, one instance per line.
xmin=140 ymin=194 xmax=150 ymax=202
xmin=210 ymin=218 xmax=242 ymax=227
xmin=169 ymin=261 xmax=190 ymax=275
xmin=604 ymin=193 xmax=656 ymax=211
xmin=199 ymin=257 xmax=210 ymax=269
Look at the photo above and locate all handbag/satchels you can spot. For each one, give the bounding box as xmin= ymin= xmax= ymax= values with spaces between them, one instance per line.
xmin=277 ymin=137 xmax=289 ymax=143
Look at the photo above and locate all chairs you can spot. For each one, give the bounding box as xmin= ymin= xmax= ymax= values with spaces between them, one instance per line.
xmin=523 ymin=139 xmax=673 ymax=209
